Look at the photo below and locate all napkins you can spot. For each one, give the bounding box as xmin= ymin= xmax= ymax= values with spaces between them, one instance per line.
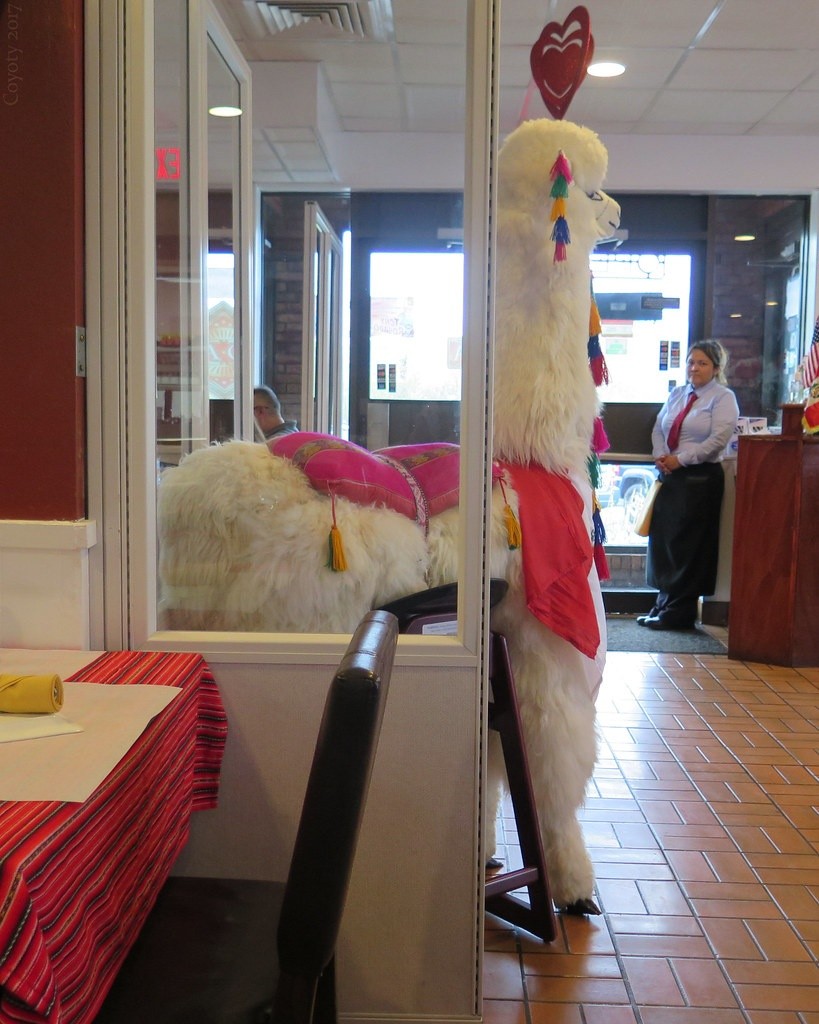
xmin=0 ymin=673 xmax=64 ymax=714
xmin=0 ymin=708 xmax=82 ymax=751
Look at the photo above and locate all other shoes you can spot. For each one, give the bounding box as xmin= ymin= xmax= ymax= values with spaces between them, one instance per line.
xmin=636 ymin=616 xmax=695 ymax=629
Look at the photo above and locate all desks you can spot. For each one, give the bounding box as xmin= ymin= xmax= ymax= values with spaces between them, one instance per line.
xmin=0 ymin=648 xmax=230 ymax=1024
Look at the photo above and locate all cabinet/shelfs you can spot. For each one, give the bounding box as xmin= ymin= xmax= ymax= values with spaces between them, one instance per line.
xmin=728 ymin=403 xmax=819 ymax=667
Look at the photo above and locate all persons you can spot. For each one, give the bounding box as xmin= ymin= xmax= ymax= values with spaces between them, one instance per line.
xmin=635 ymin=341 xmax=739 ymax=631
xmin=253 ymin=387 xmax=299 ymax=440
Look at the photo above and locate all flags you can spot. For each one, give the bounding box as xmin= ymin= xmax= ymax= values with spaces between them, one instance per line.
xmin=797 ymin=317 xmax=819 ymax=389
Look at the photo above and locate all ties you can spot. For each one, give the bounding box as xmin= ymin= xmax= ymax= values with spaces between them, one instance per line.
xmin=667 ymin=392 xmax=698 ymax=451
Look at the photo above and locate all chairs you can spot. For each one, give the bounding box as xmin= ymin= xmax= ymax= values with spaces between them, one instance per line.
xmin=93 ymin=610 xmax=400 ymax=1024
